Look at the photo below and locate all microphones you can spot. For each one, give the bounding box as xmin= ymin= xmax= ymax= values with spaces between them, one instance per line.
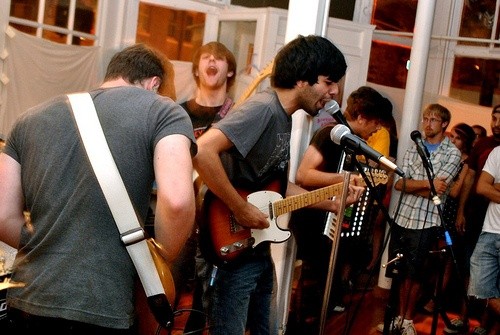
xmin=322 ymin=99 xmax=356 ymax=134
xmin=329 ymin=123 xmax=405 ymax=178
xmin=411 ymin=130 xmax=433 ymax=170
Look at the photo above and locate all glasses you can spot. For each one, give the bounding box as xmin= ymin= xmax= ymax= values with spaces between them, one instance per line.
xmin=422 ymin=116 xmax=442 ymax=124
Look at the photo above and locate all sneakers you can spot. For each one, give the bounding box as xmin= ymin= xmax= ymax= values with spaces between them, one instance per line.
xmin=376 ymin=315 xmax=401 ymax=331
xmin=401 ymin=319 xmax=417 ymax=335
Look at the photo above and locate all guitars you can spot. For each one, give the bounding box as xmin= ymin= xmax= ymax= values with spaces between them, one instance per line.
xmin=131 ymin=238 xmax=177 ymax=335
xmin=197 ymin=56 xmax=277 ymax=214
xmin=196 ymin=167 xmax=389 ymax=267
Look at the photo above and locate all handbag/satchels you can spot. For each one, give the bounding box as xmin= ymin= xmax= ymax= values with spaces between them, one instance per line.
xmin=195 ymin=253 xmax=214 ymax=310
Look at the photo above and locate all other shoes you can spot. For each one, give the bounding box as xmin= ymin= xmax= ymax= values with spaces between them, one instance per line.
xmin=445 ymin=321 xmax=470 ymax=333
xmin=471 ymin=327 xmax=489 ymax=335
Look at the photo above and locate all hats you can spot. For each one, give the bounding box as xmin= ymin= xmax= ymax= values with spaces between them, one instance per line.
xmin=455 ymin=123 xmax=477 ymax=155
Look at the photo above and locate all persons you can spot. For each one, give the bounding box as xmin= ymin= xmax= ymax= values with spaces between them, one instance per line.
xmin=172 ymin=40 xmax=239 ymax=141
xmin=486 ymin=105 xmax=500 ymax=148
xmin=0 ymin=44 xmax=194 ymax=334
xmin=375 ymin=104 xmax=462 ymax=335
xmin=191 ymin=35 xmax=365 ymax=335
xmin=290 ymin=86 xmax=389 ymax=335
xmin=419 ymin=124 xmax=485 ymax=314
xmin=467 ymin=147 xmax=500 ymax=335
